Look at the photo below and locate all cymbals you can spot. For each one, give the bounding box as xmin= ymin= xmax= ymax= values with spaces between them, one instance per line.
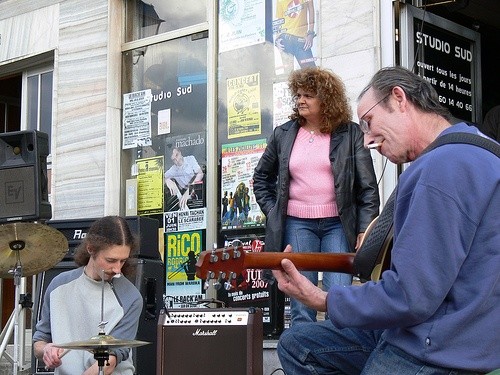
xmin=0 ymin=219 xmax=69 ymax=279
xmin=51 ymin=336 xmax=153 ymax=353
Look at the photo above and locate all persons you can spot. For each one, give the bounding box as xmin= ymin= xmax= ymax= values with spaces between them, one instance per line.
xmin=253 ymin=67 xmax=380 ymax=326
xmin=272 ymin=66 xmax=500 ymax=375
xmin=32 ymin=215 xmax=143 ymax=375
xmin=274 ymin=0 xmax=317 ymax=78
xmin=164 ymin=147 xmax=203 ymax=210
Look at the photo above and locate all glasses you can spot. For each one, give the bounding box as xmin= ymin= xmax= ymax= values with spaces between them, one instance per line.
xmin=359 ymin=93 xmax=390 ymax=134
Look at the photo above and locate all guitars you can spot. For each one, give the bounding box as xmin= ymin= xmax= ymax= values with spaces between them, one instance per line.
xmin=193 ymin=214 xmax=394 ymax=288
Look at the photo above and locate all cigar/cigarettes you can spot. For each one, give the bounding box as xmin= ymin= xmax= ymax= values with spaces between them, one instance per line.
xmin=366 ymin=143 xmax=383 ymax=149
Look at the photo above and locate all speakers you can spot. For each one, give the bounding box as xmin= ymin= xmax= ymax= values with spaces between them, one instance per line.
xmin=155 ymin=306 xmax=265 ymax=375
xmin=0 ymin=128 xmax=52 ymax=225
xmin=32 ymin=258 xmax=166 ymax=374
